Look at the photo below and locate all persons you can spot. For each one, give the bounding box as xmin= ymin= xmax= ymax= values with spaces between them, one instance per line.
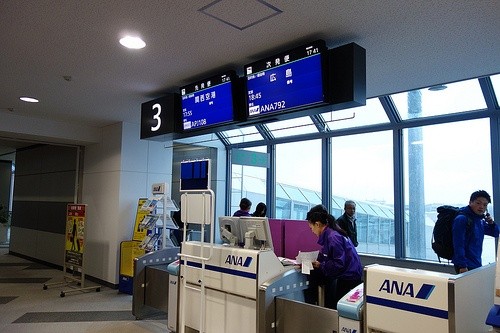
xmin=294 ymin=205 xmax=364 ymax=310
xmin=228 ymin=198 xmax=267 ymax=247
xmin=451 ymin=190 xmax=499 ymax=274
xmin=336 ymin=201 xmax=359 ymax=247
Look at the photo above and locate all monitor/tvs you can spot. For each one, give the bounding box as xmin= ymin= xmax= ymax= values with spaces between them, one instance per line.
xmin=218 ymin=217 xmax=274 ymax=250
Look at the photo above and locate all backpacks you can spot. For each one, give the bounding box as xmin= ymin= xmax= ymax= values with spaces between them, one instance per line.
xmin=431 ymin=204 xmax=476 ymax=263
